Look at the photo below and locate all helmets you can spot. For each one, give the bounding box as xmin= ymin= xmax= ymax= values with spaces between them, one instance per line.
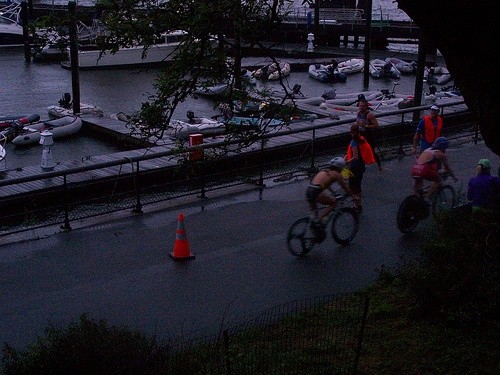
xmin=432 ymin=136 xmax=450 ymax=149
xmin=478 ymin=158 xmax=491 ymax=170
xmin=329 ymin=156 xmax=346 ymax=169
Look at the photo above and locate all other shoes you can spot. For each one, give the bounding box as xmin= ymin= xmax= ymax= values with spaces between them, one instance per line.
xmin=309 ymin=221 xmax=326 ymax=237
xmin=424 ymin=198 xmax=433 ymax=206
xmin=352 ymin=206 xmax=364 ymax=213
xmin=378 ymin=166 xmax=384 ymax=172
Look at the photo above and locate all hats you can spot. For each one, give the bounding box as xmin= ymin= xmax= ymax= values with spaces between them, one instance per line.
xmin=430 ymin=103 xmax=440 ymax=111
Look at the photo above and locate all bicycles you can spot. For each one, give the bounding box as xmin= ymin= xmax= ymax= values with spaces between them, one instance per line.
xmin=286 ymin=194 xmax=360 ymax=257
xmin=396 ymin=175 xmax=457 ymax=234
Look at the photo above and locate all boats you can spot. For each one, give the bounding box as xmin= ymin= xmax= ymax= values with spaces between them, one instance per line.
xmin=250 ymin=59 xmax=290 ymax=81
xmin=109 ymin=68 xmax=285 ymax=139
xmin=336 ymin=58 xmax=365 ymax=75
xmin=0 ymin=112 xmax=41 ymax=171
xmin=271 ymin=85 xmax=463 ymax=122
xmin=369 ymin=58 xmax=402 ymax=80
xmin=0 ymin=2 xmax=219 ymax=65
xmin=385 ymin=57 xmax=416 ymax=74
xmin=423 ymin=66 xmax=451 ymax=85
xmin=11 ymin=114 xmax=82 ymax=146
xmin=47 ymin=92 xmax=103 ymax=118
xmin=309 ymin=58 xmax=347 ymax=83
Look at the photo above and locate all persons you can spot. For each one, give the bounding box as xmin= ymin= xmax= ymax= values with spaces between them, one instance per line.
xmin=466 ymin=159 xmax=500 ymax=215
xmin=418 ymin=105 xmax=443 ymax=172
xmin=306 ymin=157 xmax=361 ymax=232
xmin=411 ymin=137 xmax=457 ymax=207
xmin=356 ymin=99 xmax=383 ymax=172
xmin=345 ymin=125 xmax=375 ymax=214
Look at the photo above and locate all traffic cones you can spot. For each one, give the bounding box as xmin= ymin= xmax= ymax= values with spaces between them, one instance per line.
xmin=167 ymin=212 xmax=196 ymax=261
